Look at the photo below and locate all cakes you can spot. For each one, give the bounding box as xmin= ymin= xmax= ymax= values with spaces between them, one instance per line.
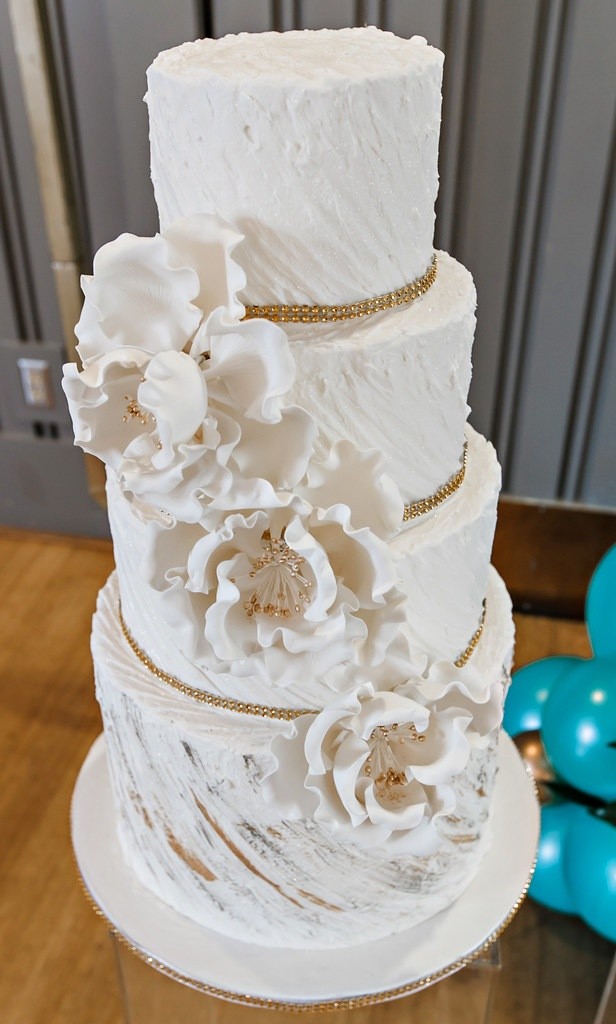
xmin=60 ymin=31 xmax=515 ymax=951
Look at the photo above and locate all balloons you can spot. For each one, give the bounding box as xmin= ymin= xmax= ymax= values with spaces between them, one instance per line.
xmin=499 ymin=544 xmax=616 ymax=944
